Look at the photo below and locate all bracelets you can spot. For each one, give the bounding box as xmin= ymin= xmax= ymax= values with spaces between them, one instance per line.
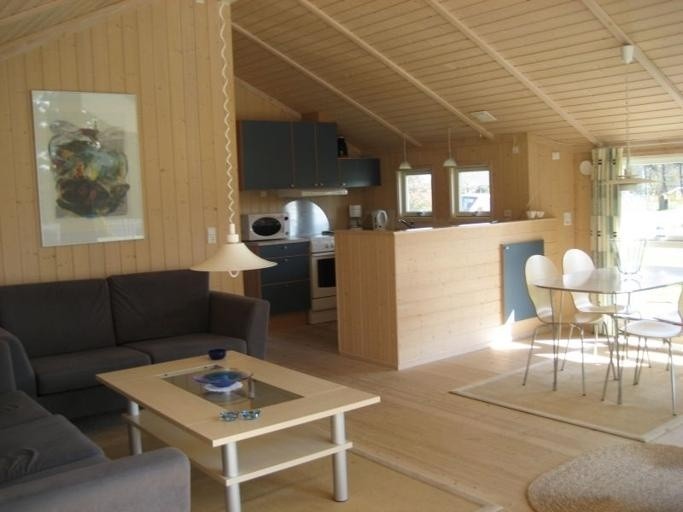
xmin=219 ymin=408 xmax=239 ymax=422
xmin=241 ymin=408 xmax=262 ymax=420
xmin=610 ymin=236 xmax=647 ymax=277
xmin=525 ymin=209 xmax=537 ymax=220
xmin=208 ymin=349 xmax=227 ymax=361
xmin=536 ymin=211 xmax=545 ymax=219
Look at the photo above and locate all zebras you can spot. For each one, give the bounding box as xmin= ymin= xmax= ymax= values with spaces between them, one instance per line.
xmin=311 ymin=251 xmax=337 ymax=299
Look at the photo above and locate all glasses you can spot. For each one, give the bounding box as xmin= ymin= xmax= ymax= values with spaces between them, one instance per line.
xmin=189 ymin=0 xmax=278 ymax=279
xmin=443 ymin=127 xmax=456 ymax=167
xmin=595 ymin=44 xmax=657 ymax=189
xmin=398 ymin=133 xmax=412 ymax=170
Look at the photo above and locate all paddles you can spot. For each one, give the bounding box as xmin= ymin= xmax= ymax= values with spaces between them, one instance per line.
xmin=448 ymin=335 xmax=683 ymax=443
xmin=527 ymin=443 xmax=683 ymax=512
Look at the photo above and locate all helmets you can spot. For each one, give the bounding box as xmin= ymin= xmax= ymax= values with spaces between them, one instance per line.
xmin=190 ymin=366 xmax=254 ymax=389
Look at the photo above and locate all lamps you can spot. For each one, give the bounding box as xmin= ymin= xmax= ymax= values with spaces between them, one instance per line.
xmin=601 ymin=288 xmax=683 ymax=416
xmin=521 ymin=248 xmax=651 ymax=395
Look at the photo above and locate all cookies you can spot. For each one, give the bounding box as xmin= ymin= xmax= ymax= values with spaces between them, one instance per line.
xmin=241 ymin=212 xmax=290 ymax=241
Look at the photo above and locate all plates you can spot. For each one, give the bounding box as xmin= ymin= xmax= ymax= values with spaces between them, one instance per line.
xmin=339 ymin=158 xmax=380 ymax=188
xmin=239 ymin=121 xmax=339 ymax=191
xmin=243 ymin=242 xmax=310 ymax=314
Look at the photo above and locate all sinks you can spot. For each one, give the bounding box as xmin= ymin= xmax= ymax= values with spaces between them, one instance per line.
xmin=0 ymin=339 xmax=191 ymax=512
xmin=1 ymin=269 xmax=270 ymax=420
